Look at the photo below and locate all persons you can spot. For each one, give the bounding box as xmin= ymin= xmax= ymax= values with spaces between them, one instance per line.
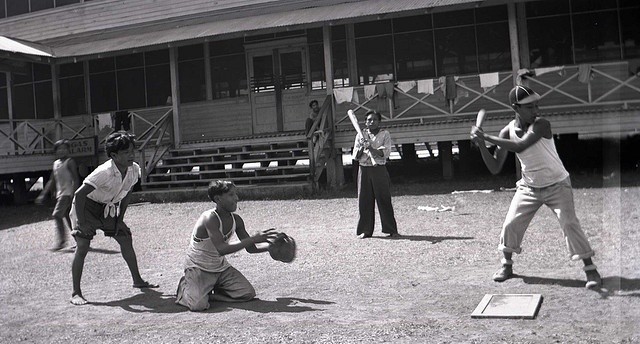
xmin=68 ymin=131 xmax=152 ymax=305
xmin=176 ymin=180 xmax=279 ymax=311
xmin=470 ymin=85 xmax=602 ymax=288
xmin=353 ymin=110 xmax=403 ymax=238
xmin=305 ymin=99 xmax=320 ymax=136
xmin=34 ymin=140 xmax=81 ymax=251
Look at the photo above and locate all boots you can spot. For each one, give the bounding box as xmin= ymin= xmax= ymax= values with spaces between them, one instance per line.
xmin=582 ymin=264 xmax=601 ymax=290
xmin=492 ymin=258 xmax=513 ymax=281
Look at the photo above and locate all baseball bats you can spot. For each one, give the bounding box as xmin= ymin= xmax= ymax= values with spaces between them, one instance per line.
xmin=347 ymin=109 xmax=377 ymax=168
xmin=472 ymin=107 xmax=487 ymax=141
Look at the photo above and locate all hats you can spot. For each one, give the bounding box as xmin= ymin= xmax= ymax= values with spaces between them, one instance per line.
xmin=509 ymin=85 xmax=541 ymax=106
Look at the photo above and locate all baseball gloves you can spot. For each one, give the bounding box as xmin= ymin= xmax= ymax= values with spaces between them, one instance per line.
xmin=268 ymin=232 xmax=296 ymax=264
